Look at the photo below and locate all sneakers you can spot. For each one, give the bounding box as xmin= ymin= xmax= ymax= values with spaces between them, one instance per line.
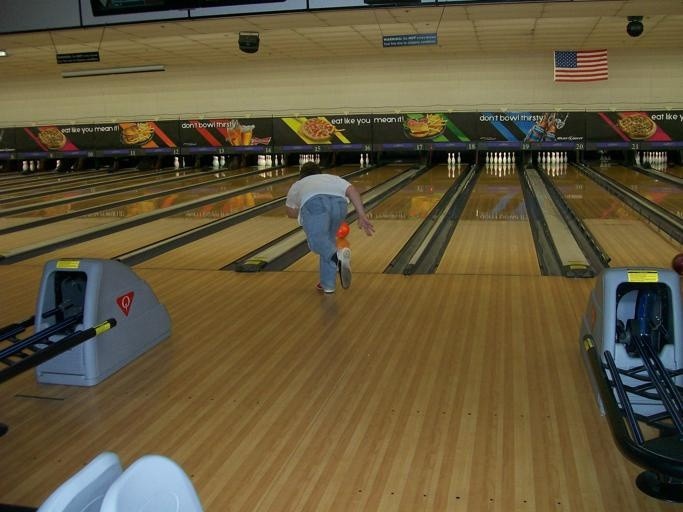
xmin=315 ymin=281 xmax=336 ymax=292
xmin=336 ymin=247 xmax=352 ymax=289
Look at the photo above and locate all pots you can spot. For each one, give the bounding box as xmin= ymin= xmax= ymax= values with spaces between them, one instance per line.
xmin=303 ymin=125 xmax=347 ymax=142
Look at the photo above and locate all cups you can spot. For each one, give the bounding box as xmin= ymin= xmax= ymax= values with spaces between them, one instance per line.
xmin=241 ymin=126 xmax=252 ymax=146
xmin=226 ymin=122 xmax=241 ymax=146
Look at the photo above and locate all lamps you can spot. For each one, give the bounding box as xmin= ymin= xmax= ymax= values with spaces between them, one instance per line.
xmin=61 ymin=65 xmax=166 ymax=78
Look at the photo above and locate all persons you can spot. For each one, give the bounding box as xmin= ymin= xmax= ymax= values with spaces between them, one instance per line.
xmin=285 ymin=162 xmax=377 ymax=294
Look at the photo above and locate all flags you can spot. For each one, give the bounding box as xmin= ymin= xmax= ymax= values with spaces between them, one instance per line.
xmin=553 ymin=47 xmax=609 ymax=83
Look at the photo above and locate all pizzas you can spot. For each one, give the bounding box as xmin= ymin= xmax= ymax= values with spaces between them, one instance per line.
xmin=303 ymin=118 xmax=335 ymax=141
xmin=38 ymin=128 xmax=67 ymax=150
xmin=618 ymin=113 xmax=657 ymax=140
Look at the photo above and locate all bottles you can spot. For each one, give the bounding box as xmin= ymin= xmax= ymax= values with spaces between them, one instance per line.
xmin=543 ymin=113 xmax=558 ymax=141
xmin=523 ymin=112 xmax=549 ymax=143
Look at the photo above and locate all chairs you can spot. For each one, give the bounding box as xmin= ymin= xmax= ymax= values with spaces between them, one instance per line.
xmin=36 ymin=451 xmax=203 ymax=512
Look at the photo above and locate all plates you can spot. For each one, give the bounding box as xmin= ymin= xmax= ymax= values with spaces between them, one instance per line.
xmin=403 ymin=122 xmax=447 ymax=142
xmin=46 ymin=134 xmax=66 ymax=151
xmin=626 ymin=120 xmax=657 ymax=141
xmin=119 ymin=127 xmax=154 ymax=147
